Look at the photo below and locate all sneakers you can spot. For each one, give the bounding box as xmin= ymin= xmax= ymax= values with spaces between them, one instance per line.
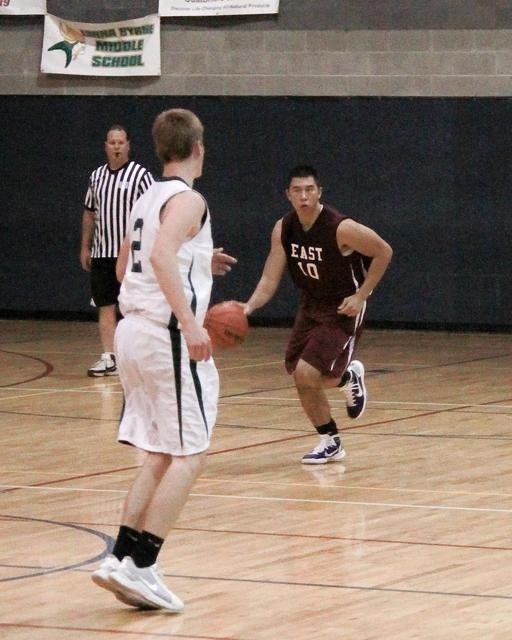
xmin=87 ymin=352 xmax=119 ymax=378
xmin=338 ymin=360 xmax=367 ymax=420
xmin=301 ymin=431 xmax=346 ymax=464
xmin=109 ymin=555 xmax=185 ymax=612
xmin=91 ymin=553 xmax=142 ymax=607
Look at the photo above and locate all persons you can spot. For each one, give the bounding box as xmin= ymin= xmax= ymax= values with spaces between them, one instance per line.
xmin=225 ymin=165 xmax=394 ymax=464
xmin=76 ymin=123 xmax=154 ymax=378
xmin=89 ymin=106 xmax=237 ymax=616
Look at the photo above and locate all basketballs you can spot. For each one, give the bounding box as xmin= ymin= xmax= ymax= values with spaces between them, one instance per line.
xmin=203 ymin=302 xmax=249 ymax=350
